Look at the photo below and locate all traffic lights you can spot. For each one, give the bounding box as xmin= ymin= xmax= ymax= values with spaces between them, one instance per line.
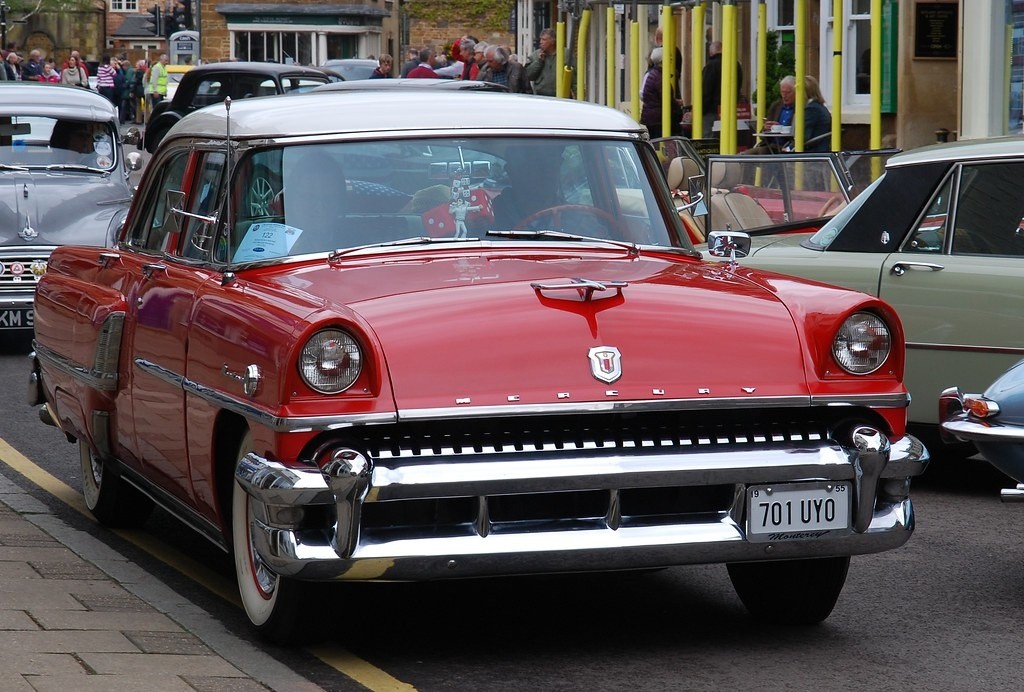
xmin=165 ymin=6 xmax=186 ymax=38
xmin=146 ymin=4 xmax=160 ymax=37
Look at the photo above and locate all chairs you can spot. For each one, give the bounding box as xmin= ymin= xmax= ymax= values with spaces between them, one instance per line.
xmin=667 ymin=152 xmax=709 ymax=237
xmin=699 ymin=162 xmax=776 ymax=238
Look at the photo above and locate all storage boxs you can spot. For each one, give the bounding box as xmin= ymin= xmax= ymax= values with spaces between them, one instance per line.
xmin=716 ymin=104 xmax=751 ymax=120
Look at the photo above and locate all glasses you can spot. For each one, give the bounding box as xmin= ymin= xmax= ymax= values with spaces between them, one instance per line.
xmin=473 ymin=51 xmax=484 ymax=55
xmin=512 ymin=154 xmax=564 ymax=175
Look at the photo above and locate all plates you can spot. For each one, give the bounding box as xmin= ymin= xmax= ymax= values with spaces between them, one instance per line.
xmin=780 ymin=132 xmax=791 ymax=134
xmin=770 ymin=131 xmax=781 ymax=134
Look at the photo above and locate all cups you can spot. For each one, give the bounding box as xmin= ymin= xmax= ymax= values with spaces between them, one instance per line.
xmin=771 ymin=124 xmax=783 ymax=131
xmin=781 ymin=125 xmax=792 ymax=132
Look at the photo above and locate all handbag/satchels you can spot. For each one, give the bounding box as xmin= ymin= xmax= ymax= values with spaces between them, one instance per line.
xmin=672 ymin=100 xmax=684 ymax=121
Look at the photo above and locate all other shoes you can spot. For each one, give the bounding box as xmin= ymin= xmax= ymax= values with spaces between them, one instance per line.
xmin=133 ymin=121 xmax=142 ymax=124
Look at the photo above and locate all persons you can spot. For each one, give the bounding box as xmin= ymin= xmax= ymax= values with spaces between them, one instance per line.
xmin=640 ymin=47 xmax=674 ymax=138
xmin=185 ymin=56 xmax=193 ymax=65
xmin=402 ymin=35 xmax=518 ymax=81
xmin=492 ymin=145 xmax=610 ymax=241
xmin=0 ymin=49 xmax=89 ymax=87
xmin=97 ymin=52 xmax=168 ymax=126
xmin=369 ymin=54 xmax=394 ymax=79
xmin=449 ymin=197 xmax=483 ymax=238
xmin=740 ymin=74 xmax=832 ymax=191
xmin=483 ymin=45 xmax=533 ymax=95
xmin=701 ymin=40 xmax=743 ymax=138
xmin=655 ymin=26 xmax=682 ymax=99
xmin=285 ymin=152 xmax=370 ymax=253
xmin=525 ymin=28 xmax=577 ymax=99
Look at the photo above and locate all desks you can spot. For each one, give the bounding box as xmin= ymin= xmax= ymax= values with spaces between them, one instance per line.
xmin=750 ymin=132 xmax=794 ymax=154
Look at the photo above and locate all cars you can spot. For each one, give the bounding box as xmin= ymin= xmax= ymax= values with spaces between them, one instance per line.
xmin=141 ymin=59 xmax=512 ymax=155
xmin=27 ymin=90 xmax=930 ymax=640
xmin=0 ymin=81 xmax=144 ymax=330
xmin=693 ymin=134 xmax=1024 ymax=424
xmin=565 ymin=136 xmax=947 ymax=245
